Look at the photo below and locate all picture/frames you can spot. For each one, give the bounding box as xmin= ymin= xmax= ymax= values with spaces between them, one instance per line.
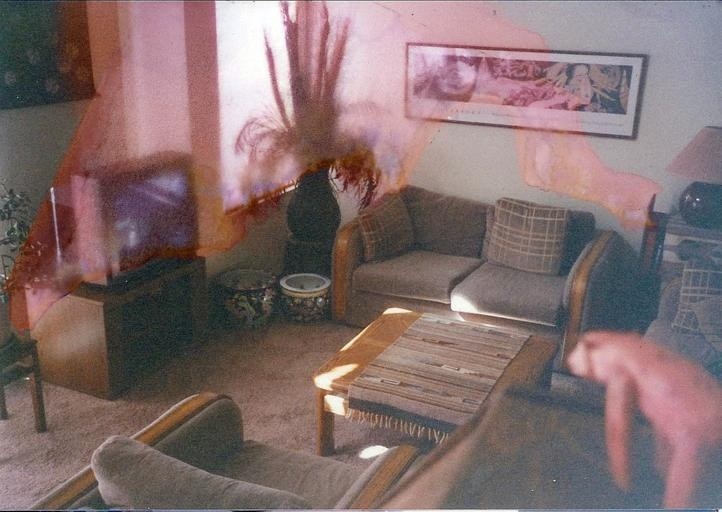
xmin=403 ymin=41 xmax=649 ymax=141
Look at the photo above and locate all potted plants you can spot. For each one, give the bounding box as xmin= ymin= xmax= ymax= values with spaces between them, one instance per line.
xmin=235 ymin=0 xmax=381 ymax=241
xmin=0 ymin=176 xmax=47 ymax=348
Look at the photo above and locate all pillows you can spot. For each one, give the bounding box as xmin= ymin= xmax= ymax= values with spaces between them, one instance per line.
xmin=355 ymin=190 xmax=416 ymax=264
xmin=487 ymin=197 xmax=569 ymax=276
xmin=90 ymin=432 xmax=312 ymax=511
xmin=671 ymin=259 xmax=722 ymax=356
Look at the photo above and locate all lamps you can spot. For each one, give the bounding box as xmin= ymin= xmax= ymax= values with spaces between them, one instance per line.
xmin=663 ymin=125 xmax=721 ymax=228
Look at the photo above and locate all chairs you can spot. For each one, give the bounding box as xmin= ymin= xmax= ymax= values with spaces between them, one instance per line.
xmin=25 ymin=391 xmax=422 ymax=511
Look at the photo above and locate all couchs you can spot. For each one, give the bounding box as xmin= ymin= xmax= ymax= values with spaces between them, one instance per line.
xmin=332 ymin=182 xmax=624 ymax=374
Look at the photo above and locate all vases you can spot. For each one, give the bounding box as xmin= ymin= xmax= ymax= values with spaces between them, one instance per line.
xmin=211 ymin=267 xmax=331 ymax=332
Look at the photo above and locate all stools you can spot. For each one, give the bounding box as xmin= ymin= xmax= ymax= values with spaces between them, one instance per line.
xmin=0 ymin=338 xmax=46 ymax=433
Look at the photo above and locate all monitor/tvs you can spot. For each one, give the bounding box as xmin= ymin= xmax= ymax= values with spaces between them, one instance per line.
xmin=69 ymin=149 xmax=198 ymax=287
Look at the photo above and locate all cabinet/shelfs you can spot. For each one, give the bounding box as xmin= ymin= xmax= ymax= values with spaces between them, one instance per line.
xmin=17 ymin=256 xmax=212 ymax=402
